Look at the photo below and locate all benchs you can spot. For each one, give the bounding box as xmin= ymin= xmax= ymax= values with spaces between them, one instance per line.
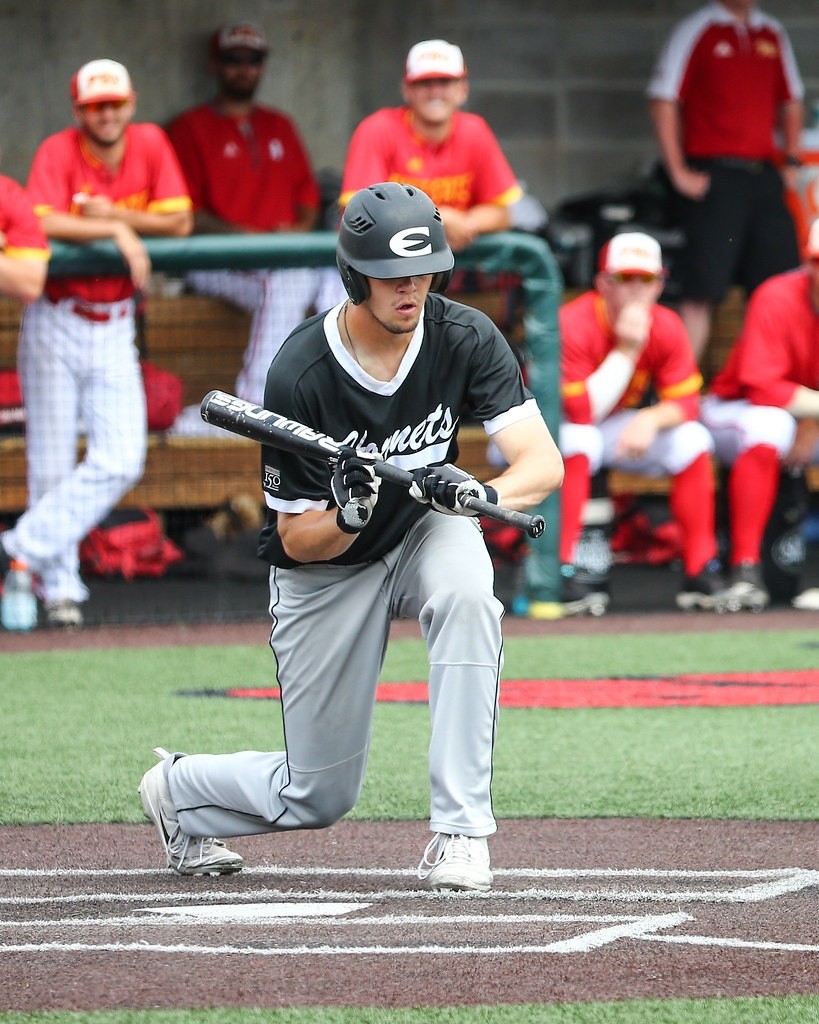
xmin=0 ymin=285 xmax=742 ymax=509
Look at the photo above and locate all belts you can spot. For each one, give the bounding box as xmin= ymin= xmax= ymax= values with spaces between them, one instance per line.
xmin=45 ymin=296 xmax=133 ymax=324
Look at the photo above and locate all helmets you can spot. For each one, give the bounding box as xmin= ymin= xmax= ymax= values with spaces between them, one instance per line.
xmin=336 ymin=182 xmax=457 ymax=307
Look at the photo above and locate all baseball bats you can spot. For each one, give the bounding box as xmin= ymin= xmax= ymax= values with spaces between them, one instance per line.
xmin=199 ymin=389 xmax=548 ymax=540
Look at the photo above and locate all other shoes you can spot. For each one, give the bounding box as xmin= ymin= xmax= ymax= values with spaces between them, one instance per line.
xmin=44 ymin=600 xmax=85 ymax=631
xmin=1 ymin=563 xmax=39 ymax=632
xmin=790 ymin=587 xmax=819 ymax=612
xmin=674 ymin=560 xmax=754 ymax=615
xmin=528 ymin=576 xmax=612 ymax=620
xmin=730 ymin=563 xmax=772 ymax=611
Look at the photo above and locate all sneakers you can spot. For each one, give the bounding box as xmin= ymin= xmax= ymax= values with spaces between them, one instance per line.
xmin=137 ymin=745 xmax=246 ymax=875
xmin=415 ymin=827 xmax=496 ymax=892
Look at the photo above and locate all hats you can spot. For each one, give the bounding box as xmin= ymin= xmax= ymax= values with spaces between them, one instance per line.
xmin=72 ymin=59 xmax=133 ymax=106
xmin=407 ymin=36 xmax=468 ymax=83
xmin=603 ymin=232 xmax=662 ymax=279
xmin=805 ymin=218 xmax=819 ymax=256
xmin=214 ymin=21 xmax=275 ymax=51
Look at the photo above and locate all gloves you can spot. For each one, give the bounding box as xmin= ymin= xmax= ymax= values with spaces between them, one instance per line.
xmin=408 ymin=462 xmax=498 ymax=518
xmin=331 ymin=443 xmax=384 ymax=534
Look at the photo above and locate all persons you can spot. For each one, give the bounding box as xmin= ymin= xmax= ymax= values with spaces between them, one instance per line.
xmin=484 ymin=230 xmax=757 ymax=619
xmin=138 ymin=181 xmax=563 ymax=891
xmin=0 ymin=0 xmax=818 ymax=632
xmin=0 ymin=57 xmax=196 ymax=631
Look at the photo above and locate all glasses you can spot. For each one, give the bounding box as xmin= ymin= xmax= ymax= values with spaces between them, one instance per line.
xmin=218 ymin=52 xmax=265 ymax=66
